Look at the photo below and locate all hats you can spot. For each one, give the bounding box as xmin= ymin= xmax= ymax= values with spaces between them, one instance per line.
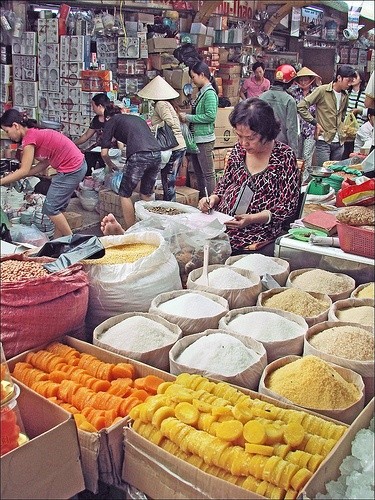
xmin=295 ymin=67 xmax=322 ymax=80
xmin=137 ymin=75 xmax=180 ymax=100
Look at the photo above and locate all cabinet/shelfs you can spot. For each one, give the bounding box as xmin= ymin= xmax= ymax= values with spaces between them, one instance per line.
xmin=260 ymin=51 xmax=298 ymax=82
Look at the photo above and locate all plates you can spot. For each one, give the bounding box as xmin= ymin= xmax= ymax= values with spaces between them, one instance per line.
xmin=288 ymin=228 xmax=328 ymax=242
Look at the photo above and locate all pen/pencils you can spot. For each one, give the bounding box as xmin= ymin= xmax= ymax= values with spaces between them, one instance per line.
xmin=205 ymin=187 xmax=210 ymax=214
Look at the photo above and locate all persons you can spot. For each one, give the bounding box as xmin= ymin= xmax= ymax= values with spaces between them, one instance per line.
xmin=238 ymin=61 xmax=271 ymax=100
xmin=257 ymin=64 xmax=299 ymax=159
xmin=178 ymin=62 xmax=219 ymax=200
xmin=1 ymin=108 xmax=88 ymax=240
xmin=137 ymin=75 xmax=187 ymax=203
xmin=296 ymin=65 xmax=357 ymax=167
xmin=73 ymin=92 xmax=114 ymax=176
xmin=102 ymin=101 xmax=162 ymax=230
xmin=100 ymin=96 xmax=301 ymax=258
xmin=342 ymin=68 xmax=375 ymax=177
xmin=295 ymin=66 xmax=322 ymax=168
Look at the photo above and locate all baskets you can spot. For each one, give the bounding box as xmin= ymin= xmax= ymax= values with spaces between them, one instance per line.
xmin=335 ymin=220 xmax=375 ymax=259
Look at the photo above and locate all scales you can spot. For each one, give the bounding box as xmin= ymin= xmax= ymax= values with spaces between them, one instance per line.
xmin=179 ymin=84 xmax=193 ymax=109
xmin=305 ymin=166 xmax=334 ymax=194
xmin=41 ymin=121 xmax=62 ymax=130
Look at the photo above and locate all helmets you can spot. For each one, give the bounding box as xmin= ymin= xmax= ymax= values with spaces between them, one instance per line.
xmin=274 ymin=64 xmax=298 ymax=83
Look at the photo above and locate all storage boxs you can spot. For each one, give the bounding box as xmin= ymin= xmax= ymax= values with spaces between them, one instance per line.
xmin=6 ymin=334 xmax=178 ymax=500
xmin=0 ymin=376 xmax=86 ymax=500
xmin=121 ymin=376 xmax=349 ymax=500
xmin=0 ymin=12 xmax=245 ymax=230
xmin=297 ymin=395 xmax=375 ymax=499
xmin=336 ymin=221 xmax=375 ymax=258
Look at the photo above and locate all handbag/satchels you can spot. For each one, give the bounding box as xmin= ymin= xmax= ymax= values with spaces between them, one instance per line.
xmin=156 ymin=120 xmax=179 ymax=151
xmin=111 ymin=169 xmax=123 ymax=194
xmin=180 ymin=122 xmax=199 ymax=154
xmin=339 ymin=112 xmax=359 ymax=141
